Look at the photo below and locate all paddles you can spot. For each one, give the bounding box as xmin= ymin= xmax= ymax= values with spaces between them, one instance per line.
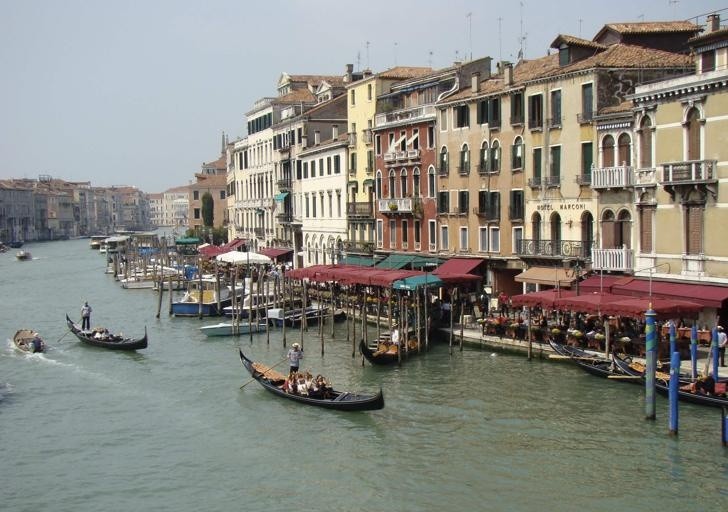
xmin=548 ymin=354 xmax=605 ymax=363
xmin=608 ymin=375 xmax=642 ymax=379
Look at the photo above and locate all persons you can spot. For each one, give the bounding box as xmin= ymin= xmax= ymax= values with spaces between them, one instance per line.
xmin=285 ymin=342 xmax=304 ymax=375
xmin=284 ymin=367 xmax=337 ymax=400
xmin=32 ymin=333 xmax=45 ymax=354
xmin=79 ymin=298 xmax=93 ymax=330
xmin=313 ymin=282 xmax=479 ymax=329
xmin=87 ymin=325 xmax=124 ymax=342
xmin=715 ymin=323 xmax=727 ymax=368
xmin=480 ymin=291 xmax=687 ymax=359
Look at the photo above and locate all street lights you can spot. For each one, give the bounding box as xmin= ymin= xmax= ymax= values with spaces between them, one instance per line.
xmin=561 ymin=255 xmax=593 ymax=297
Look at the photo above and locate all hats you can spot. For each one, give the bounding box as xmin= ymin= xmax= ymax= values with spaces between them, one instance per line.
xmin=33 ymin=333 xmax=39 ymax=337
xmin=392 ymin=323 xmax=398 ymax=326
xmin=92 ymin=327 xmax=102 ymax=331
xmin=292 ymin=343 xmax=300 ymax=348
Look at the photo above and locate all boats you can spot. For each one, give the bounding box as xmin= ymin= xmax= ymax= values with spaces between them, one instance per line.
xmin=358 ymin=317 xmax=431 ymax=366
xmin=89 ymin=230 xmax=344 ymax=338
xmin=238 ymin=347 xmax=384 ymax=410
xmin=65 ymin=313 xmax=147 ymax=350
xmin=15 ymin=251 xmax=32 ymax=259
xmin=14 ymin=330 xmax=44 ymax=354
xmin=546 ymin=335 xmax=728 ymax=409
